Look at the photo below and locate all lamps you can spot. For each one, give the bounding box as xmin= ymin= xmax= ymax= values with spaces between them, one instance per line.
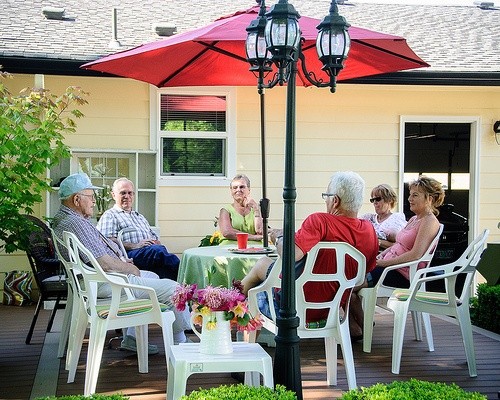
xmin=494 ymin=121 xmax=500 ymax=145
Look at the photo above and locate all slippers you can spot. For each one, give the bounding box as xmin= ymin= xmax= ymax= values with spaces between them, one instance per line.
xmin=349 ymin=332 xmax=363 ymax=341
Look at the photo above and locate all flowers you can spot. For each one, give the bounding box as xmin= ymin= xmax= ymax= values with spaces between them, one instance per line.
xmin=172 ymin=278 xmax=263 ymax=336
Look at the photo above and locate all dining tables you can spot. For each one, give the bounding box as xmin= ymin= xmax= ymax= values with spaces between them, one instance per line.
xmin=177 ymin=244 xmax=280 ymax=292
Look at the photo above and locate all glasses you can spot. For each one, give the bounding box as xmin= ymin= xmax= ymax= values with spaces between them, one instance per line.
xmin=370 ymin=196 xmax=384 ymax=203
xmin=322 ymin=193 xmax=337 ymax=199
xmin=78 ymin=193 xmax=95 ymax=200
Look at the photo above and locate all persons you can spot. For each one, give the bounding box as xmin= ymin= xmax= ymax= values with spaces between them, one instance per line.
xmin=342 ymin=177 xmax=445 ymax=341
xmin=219 ymin=175 xmax=263 ymax=245
xmin=240 ymin=172 xmax=379 ymax=324
xmin=96 ymin=178 xmax=198 ymax=334
xmin=50 ymin=173 xmax=194 ymax=355
xmin=358 ymin=184 xmax=408 ymax=249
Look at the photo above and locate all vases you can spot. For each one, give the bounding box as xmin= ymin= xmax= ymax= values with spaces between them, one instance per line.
xmin=191 ymin=310 xmax=233 ymax=355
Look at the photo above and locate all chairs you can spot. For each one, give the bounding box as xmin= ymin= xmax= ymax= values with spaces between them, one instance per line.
xmin=17 ymin=223 xmax=489 ymax=400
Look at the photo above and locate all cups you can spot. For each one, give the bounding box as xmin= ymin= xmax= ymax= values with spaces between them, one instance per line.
xmin=236 ymin=233 xmax=248 ymax=249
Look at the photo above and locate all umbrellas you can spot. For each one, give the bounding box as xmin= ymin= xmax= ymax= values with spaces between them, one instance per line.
xmin=80 ymin=6 xmax=431 ymax=248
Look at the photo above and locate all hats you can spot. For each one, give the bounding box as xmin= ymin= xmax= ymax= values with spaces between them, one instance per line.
xmin=58 ymin=173 xmax=104 ymax=196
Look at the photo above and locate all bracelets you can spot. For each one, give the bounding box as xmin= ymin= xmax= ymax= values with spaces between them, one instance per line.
xmin=379 ymin=238 xmax=381 ymax=246
xmin=275 ymin=235 xmax=283 ymax=247
xmin=254 ymin=210 xmax=260 ymax=217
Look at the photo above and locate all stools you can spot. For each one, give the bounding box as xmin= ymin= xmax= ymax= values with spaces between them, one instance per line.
xmin=165 ymin=343 xmax=274 ymax=400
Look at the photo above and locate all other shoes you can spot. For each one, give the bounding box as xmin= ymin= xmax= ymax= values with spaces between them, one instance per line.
xmin=120 ymin=337 xmax=157 ymax=353
xmin=184 ymin=325 xmax=202 ymax=335
xmin=183 ymin=336 xmax=193 ymax=343
